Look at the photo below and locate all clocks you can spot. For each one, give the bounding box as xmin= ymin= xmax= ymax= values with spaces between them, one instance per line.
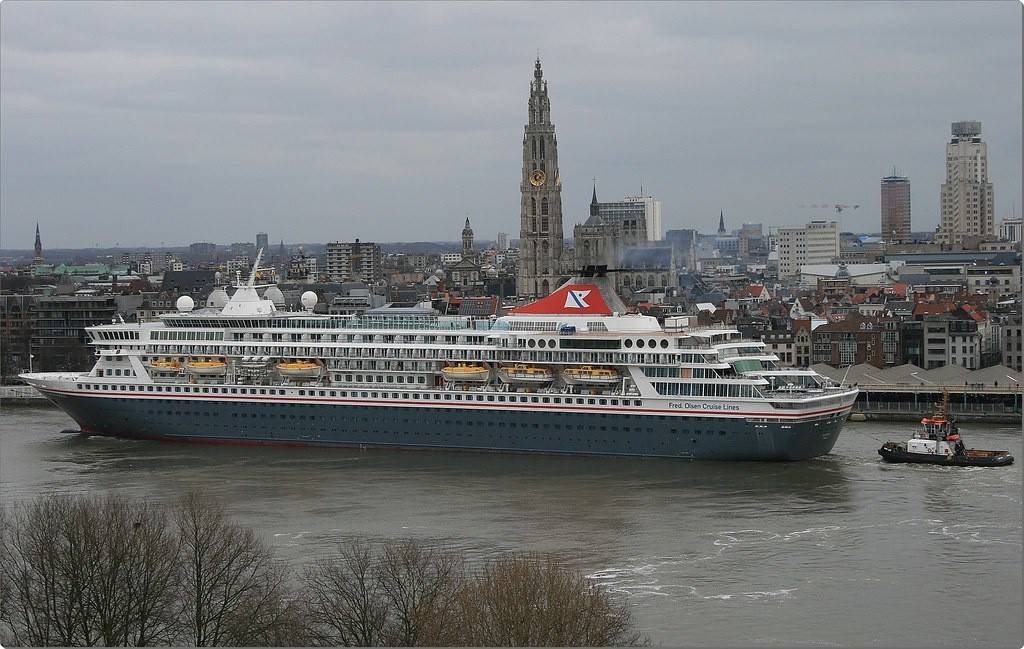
xmin=555 ymin=168 xmax=560 ymax=186
xmin=529 ymin=169 xmax=546 ymax=187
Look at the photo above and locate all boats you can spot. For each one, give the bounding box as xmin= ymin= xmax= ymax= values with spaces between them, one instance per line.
xmin=276 ymin=360 xmax=323 ymax=378
xmin=149 ymin=362 xmax=181 ymax=374
xmin=187 ymin=360 xmax=227 ymax=376
xmin=878 ymin=382 xmax=1015 ymax=466
xmin=495 ymin=364 xmax=557 ymax=387
xmin=440 ymin=363 xmax=490 ymax=383
xmin=558 ymin=365 xmax=620 ymax=387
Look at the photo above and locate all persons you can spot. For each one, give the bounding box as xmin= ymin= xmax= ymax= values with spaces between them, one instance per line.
xmin=849 ymin=382 xmax=857 ymax=388
xmin=835 ymin=383 xmax=840 ymax=387
xmin=955 ymin=440 xmax=964 ymax=451
xmin=935 ymin=426 xmax=959 ymax=437
xmin=808 ymin=382 xmax=818 ymax=388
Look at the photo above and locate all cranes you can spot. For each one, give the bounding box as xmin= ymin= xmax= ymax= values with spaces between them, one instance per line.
xmin=796 ymin=203 xmax=861 ymax=223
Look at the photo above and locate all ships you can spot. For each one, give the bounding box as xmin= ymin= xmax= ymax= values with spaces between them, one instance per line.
xmin=15 ymin=243 xmax=865 ymax=466
